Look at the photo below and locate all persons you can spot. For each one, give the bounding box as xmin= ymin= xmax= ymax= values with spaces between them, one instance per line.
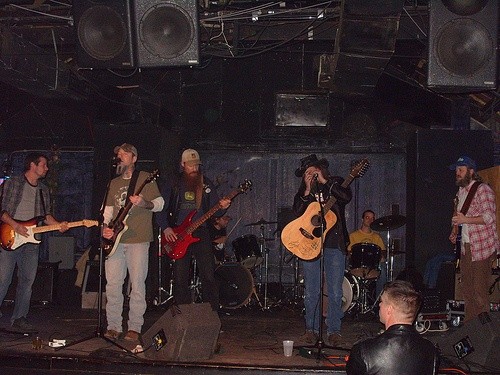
xmin=0 ymin=152 xmax=69 ymax=334
xmin=293 ymin=153 xmax=352 ymax=346
xmin=345 ymin=209 xmax=388 ymax=317
xmin=158 ymin=147 xmax=231 ymax=314
xmin=342 ymin=280 xmax=441 ymax=375
xmin=205 ymin=213 xmax=229 ymax=266
xmin=447 ymin=154 xmax=497 ymax=325
xmin=92 ymin=141 xmax=165 ymax=342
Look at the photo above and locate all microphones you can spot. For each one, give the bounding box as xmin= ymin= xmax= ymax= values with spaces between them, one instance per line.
xmin=112 ymin=158 xmax=121 ymax=163
xmin=312 ymin=172 xmax=318 ymax=178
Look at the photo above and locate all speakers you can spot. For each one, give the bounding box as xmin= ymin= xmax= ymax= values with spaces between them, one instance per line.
xmin=427 ymin=0 xmax=499 ymax=92
xmin=142 ymin=302 xmax=222 ymax=361
xmin=28 ymin=259 xmax=59 ymax=307
xmin=433 ymin=311 xmax=500 ymax=372
xmin=72 ymin=0 xmax=201 ymax=70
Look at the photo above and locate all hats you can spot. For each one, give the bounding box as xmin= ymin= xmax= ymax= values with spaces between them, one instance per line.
xmin=449 ymin=154 xmax=477 ymax=171
xmin=182 ymin=148 xmax=203 ymax=167
xmin=117 ymin=143 xmax=138 ymax=156
xmin=295 ymin=154 xmax=330 ymax=178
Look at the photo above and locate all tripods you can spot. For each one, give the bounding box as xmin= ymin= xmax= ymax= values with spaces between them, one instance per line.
xmin=294 ymin=178 xmax=351 ymax=360
xmin=53 ymin=165 xmax=141 ymax=359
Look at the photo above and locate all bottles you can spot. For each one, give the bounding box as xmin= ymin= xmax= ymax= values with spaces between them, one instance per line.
xmin=32 ymin=336 xmax=42 ymax=349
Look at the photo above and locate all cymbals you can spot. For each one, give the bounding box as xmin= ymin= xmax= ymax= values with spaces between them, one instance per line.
xmin=256 ymin=237 xmax=275 ymax=242
xmin=370 ymin=214 xmax=408 ymax=231
xmin=243 ymin=217 xmax=279 ymax=226
xmin=383 ymin=249 xmax=407 ymax=257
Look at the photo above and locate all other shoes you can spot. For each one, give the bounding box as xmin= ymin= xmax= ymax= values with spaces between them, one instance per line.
xmin=104 ymin=329 xmax=139 ymax=342
xmin=306 ymin=333 xmax=349 ymax=348
xmin=11 ymin=318 xmax=34 ymax=330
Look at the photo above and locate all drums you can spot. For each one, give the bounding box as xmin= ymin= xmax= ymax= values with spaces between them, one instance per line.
xmin=231 ymin=234 xmax=264 ymax=267
xmin=321 ymin=269 xmax=361 ymax=318
xmin=349 ymin=241 xmax=382 ymax=279
xmin=212 ymin=262 xmax=255 ymax=310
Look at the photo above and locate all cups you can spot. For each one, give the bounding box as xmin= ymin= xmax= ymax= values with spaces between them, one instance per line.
xmin=282 ymin=340 xmax=295 ymax=357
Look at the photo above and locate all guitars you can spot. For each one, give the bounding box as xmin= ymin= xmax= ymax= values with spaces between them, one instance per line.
xmin=0 ymin=216 xmax=99 ymax=252
xmin=161 ymin=177 xmax=253 ymax=261
xmin=447 ymin=196 xmax=460 ymax=268
xmin=96 ymin=167 xmax=160 ymax=257
xmin=280 ymin=157 xmax=371 ymax=261
